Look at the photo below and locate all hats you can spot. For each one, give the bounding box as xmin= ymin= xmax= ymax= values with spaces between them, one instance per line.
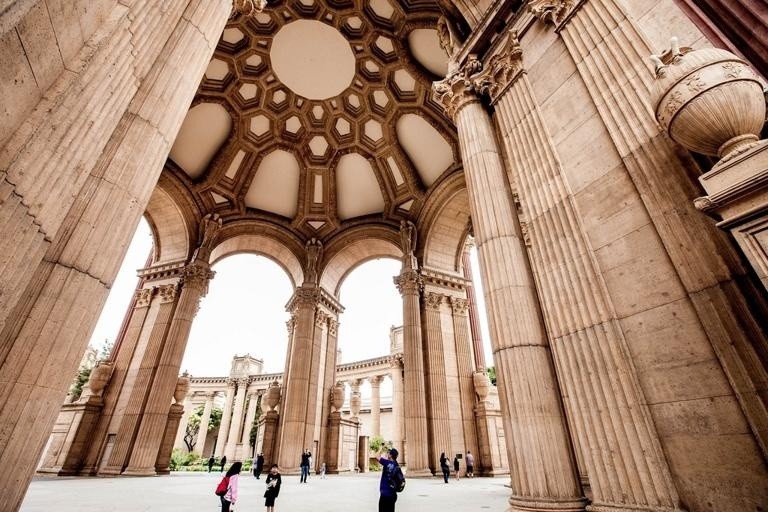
xmin=388 ymin=449 xmax=398 ymax=456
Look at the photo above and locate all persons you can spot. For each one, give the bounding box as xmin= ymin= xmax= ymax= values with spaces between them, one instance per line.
xmin=377 ymin=447 xmax=403 ymax=512
xmin=440 ymin=452 xmax=451 ymax=483
xmin=220 ymin=456 xmax=226 ymax=473
xmin=465 ymin=450 xmax=474 ymax=477
xmin=221 ymin=461 xmax=243 ymax=512
xmin=256 ymin=452 xmax=265 ymax=479
xmin=208 ymin=455 xmax=214 ymax=473
xmin=264 ymin=463 xmax=282 ymax=512
xmin=254 ymin=453 xmax=259 ymax=476
xmin=454 ymin=457 xmax=460 ymax=481
xmin=300 ymin=448 xmax=326 ymax=484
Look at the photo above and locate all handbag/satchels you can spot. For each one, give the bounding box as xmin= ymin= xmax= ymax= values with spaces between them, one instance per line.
xmin=215 ymin=477 xmax=229 ymax=495
xmin=389 ymin=466 xmax=406 ymax=492
xmin=445 ymin=460 xmax=450 ymax=467
